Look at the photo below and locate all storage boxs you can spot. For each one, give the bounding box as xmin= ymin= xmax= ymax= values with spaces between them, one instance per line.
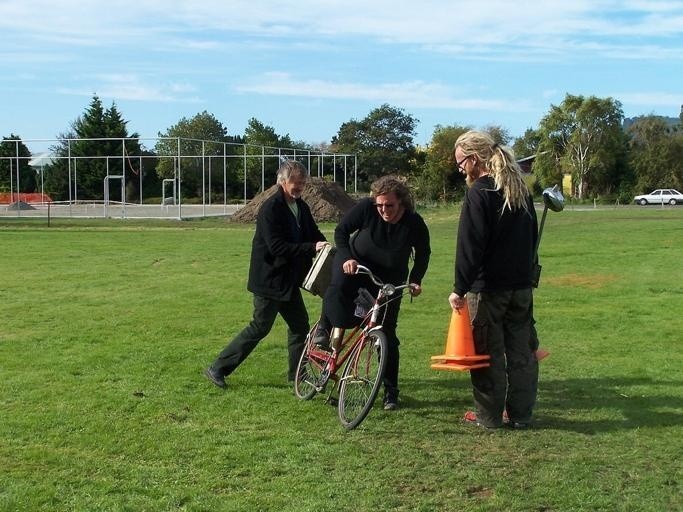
xmin=300 ymin=243 xmax=339 ymax=298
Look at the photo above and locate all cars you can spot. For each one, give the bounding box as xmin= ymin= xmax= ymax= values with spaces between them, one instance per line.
xmin=631 ymin=187 xmax=682 ymax=207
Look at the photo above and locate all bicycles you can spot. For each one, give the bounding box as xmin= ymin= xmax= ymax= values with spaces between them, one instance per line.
xmin=294 ymin=261 xmax=419 ymax=430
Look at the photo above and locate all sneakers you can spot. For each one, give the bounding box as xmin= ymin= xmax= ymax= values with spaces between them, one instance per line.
xmin=384 ymin=398 xmax=396 ymax=410
xmin=466 ymin=412 xmax=526 ymax=427
xmin=313 ymin=324 xmax=328 ymax=344
xmin=206 ymin=367 xmax=223 ymax=386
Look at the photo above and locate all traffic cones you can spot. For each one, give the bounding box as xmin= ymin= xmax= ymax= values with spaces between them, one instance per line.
xmin=424 ymin=292 xmax=489 ymax=375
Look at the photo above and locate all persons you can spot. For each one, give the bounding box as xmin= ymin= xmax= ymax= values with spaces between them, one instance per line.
xmin=203 ymin=158 xmax=329 ymax=388
xmin=448 ymin=131 xmax=541 ymax=431
xmin=313 ymin=174 xmax=431 ymax=411
